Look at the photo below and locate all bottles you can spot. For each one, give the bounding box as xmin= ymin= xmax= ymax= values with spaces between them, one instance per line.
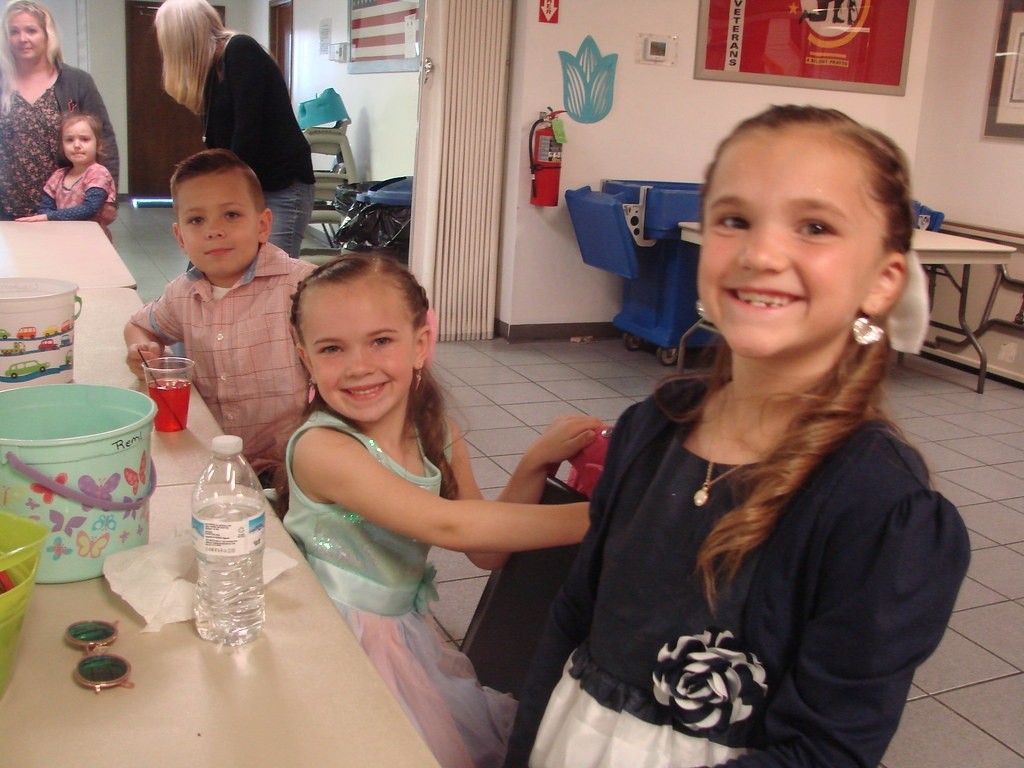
xmin=190 ymin=434 xmax=266 ymax=648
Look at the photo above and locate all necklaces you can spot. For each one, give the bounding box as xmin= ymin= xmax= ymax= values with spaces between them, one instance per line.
xmin=694 ymin=392 xmax=755 ymax=507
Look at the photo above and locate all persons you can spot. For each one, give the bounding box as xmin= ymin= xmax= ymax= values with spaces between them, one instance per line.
xmin=14 ymin=114 xmax=116 ymax=242
xmin=500 ymin=104 xmax=971 ymax=768
xmin=282 ymin=254 xmax=601 ymax=767
xmin=124 ymin=150 xmax=319 ymax=520
xmin=0 ymin=0 xmax=119 ymax=242
xmin=155 ymin=0 xmax=317 ymax=260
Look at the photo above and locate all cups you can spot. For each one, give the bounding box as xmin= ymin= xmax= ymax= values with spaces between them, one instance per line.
xmin=141 ymin=357 xmax=195 ymax=432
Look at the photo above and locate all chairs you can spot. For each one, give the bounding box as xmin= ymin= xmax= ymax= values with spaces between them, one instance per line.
xmin=459 ymin=422 xmax=614 ymax=703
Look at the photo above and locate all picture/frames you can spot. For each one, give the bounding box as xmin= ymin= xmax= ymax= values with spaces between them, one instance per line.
xmin=693 ymin=0 xmax=919 ymax=98
xmin=347 ymin=0 xmax=425 ymax=74
xmin=982 ymin=0 xmax=1024 ymax=142
xmin=917 ymin=222 xmax=1024 ymax=391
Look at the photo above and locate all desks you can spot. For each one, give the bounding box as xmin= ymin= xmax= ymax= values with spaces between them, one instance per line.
xmin=0 ymin=221 xmax=138 ymax=288
xmin=674 ymin=220 xmax=1018 ymax=394
xmin=0 ymin=483 xmax=444 ymax=768
xmin=69 ymin=287 xmax=243 ymax=486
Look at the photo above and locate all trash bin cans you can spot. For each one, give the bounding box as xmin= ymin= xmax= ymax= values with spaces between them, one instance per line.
xmin=356 ymin=175 xmax=413 ymax=266
xmin=336 ymin=181 xmax=383 ymax=255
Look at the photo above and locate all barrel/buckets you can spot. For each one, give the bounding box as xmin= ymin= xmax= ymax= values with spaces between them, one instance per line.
xmin=1 ymin=277 xmax=82 ymax=390
xmin=1 ymin=512 xmax=48 ymax=701
xmin=1 ymin=382 xmax=158 ymax=586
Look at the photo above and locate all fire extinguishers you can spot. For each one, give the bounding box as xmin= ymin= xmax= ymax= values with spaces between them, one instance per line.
xmin=529 ymin=106 xmax=568 ymax=206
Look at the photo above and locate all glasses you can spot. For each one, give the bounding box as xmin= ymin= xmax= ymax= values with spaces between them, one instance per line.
xmin=65 ymin=618 xmax=134 ymax=692
